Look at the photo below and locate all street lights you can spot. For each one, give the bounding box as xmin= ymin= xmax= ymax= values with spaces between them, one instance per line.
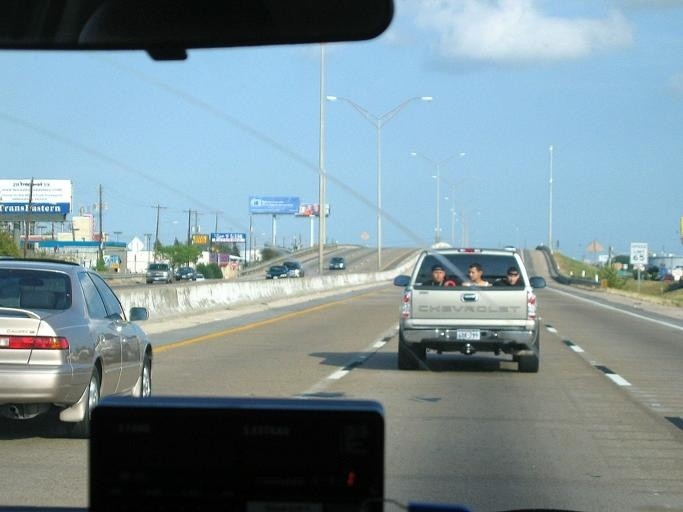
xmin=324 ymin=94 xmax=432 ymax=271
xmin=410 ymin=152 xmax=465 ymax=244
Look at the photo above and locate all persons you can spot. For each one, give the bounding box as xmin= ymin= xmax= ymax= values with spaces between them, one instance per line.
xmin=493 ymin=266 xmax=522 ymax=287
xmin=458 ymin=262 xmax=492 ymax=287
xmin=421 ymin=265 xmax=451 ymax=287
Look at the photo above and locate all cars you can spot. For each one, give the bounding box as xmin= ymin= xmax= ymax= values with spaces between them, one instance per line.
xmin=195 ymin=274 xmax=204 ymax=281
xmin=175 ymin=267 xmax=195 ymax=281
xmin=282 ymin=261 xmax=304 ymax=278
xmin=328 ymin=257 xmax=344 ymax=270
xmin=265 ymin=265 xmax=289 ymax=279
xmin=0 ymin=258 xmax=152 ymax=438
xmin=392 ymin=246 xmax=546 ymax=373
xmin=145 ymin=263 xmax=172 ymax=284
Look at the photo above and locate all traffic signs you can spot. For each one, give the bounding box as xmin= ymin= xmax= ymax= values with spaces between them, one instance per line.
xmin=629 ymin=242 xmax=647 ymax=266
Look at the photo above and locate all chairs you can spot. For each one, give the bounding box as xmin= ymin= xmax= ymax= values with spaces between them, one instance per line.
xmin=20 ymin=289 xmax=56 ymax=309
